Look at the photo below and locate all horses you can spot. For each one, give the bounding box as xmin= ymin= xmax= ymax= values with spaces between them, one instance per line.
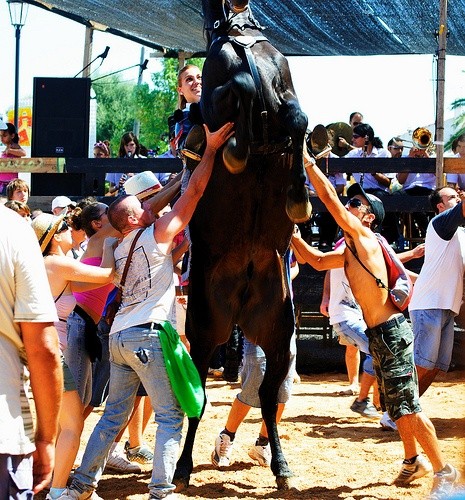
xmin=171 ymin=0 xmax=313 ymax=493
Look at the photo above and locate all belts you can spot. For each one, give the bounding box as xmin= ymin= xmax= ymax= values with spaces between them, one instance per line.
xmin=132 ymin=323 xmax=164 ymax=330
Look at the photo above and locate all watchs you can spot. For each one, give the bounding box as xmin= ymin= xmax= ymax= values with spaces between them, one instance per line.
xmin=302 ymin=157 xmax=318 ymax=169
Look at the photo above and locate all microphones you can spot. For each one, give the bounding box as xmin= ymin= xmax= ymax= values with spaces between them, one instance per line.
xmin=140 ymin=59 xmax=149 ymax=76
xmin=100 ymin=46 xmax=110 ymax=67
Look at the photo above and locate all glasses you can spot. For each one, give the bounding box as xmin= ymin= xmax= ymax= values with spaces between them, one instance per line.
xmin=350 ymin=121 xmax=361 ymax=126
xmin=94 ymin=142 xmax=108 ymax=154
xmin=353 ymin=135 xmax=361 ymax=138
xmin=389 ymin=145 xmax=403 ymax=150
xmin=57 ymin=222 xmax=68 ymax=233
xmin=96 ymin=207 xmax=108 ymax=220
xmin=347 ymin=198 xmax=370 ymax=214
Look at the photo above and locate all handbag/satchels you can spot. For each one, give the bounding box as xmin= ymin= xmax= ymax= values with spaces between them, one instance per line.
xmin=376 ymin=231 xmax=413 ymax=311
xmin=105 ymin=288 xmax=121 ymax=326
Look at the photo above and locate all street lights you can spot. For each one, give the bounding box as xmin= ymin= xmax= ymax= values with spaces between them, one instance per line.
xmin=6 ymin=0 xmax=29 ymax=135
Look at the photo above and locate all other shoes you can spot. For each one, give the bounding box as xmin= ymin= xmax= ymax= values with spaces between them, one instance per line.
xmin=339 ymin=384 xmax=360 ymax=395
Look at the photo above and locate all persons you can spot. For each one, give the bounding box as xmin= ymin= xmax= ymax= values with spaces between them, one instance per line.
xmin=319 ymin=237 xmax=361 ymax=395
xmin=328 ymin=237 xmax=381 ymax=417
xmin=62 ymin=116 xmax=238 ymax=500
xmin=0 ymin=120 xmax=190 ymax=473
xmin=302 ymin=112 xmax=465 ymax=250
xmin=211 ymin=223 xmax=307 ymax=468
xmin=292 ymin=135 xmax=460 ymax=500
xmin=0 ymin=202 xmax=60 ymax=499
xmin=172 ymin=64 xmax=217 ymax=193
xmin=379 ymin=184 xmax=465 ymax=431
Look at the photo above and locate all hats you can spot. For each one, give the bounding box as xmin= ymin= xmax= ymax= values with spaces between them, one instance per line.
xmin=51 ymin=196 xmax=76 ymax=212
xmin=31 ymin=206 xmax=68 ymax=254
xmin=347 ymin=183 xmax=385 ymax=230
xmin=123 ymin=170 xmax=164 ymax=200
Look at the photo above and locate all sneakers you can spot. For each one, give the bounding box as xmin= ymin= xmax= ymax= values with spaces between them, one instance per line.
xmin=148 ymin=492 xmax=181 ymax=500
xmin=102 ymin=451 xmax=141 ymax=474
xmin=350 ymin=397 xmax=383 ymax=419
xmin=124 ymin=442 xmax=154 ymax=464
xmin=212 ymin=434 xmax=233 ymax=470
xmin=247 ymin=441 xmax=272 ymax=466
xmin=380 ymin=411 xmax=398 ymax=431
xmin=61 ymin=484 xmax=104 ymax=500
xmin=427 ymin=463 xmax=461 ymax=500
xmin=393 ymin=454 xmax=431 ymax=484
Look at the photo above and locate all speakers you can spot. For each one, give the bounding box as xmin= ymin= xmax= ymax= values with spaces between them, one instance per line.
xmin=31 ymin=77 xmax=98 ymax=196
